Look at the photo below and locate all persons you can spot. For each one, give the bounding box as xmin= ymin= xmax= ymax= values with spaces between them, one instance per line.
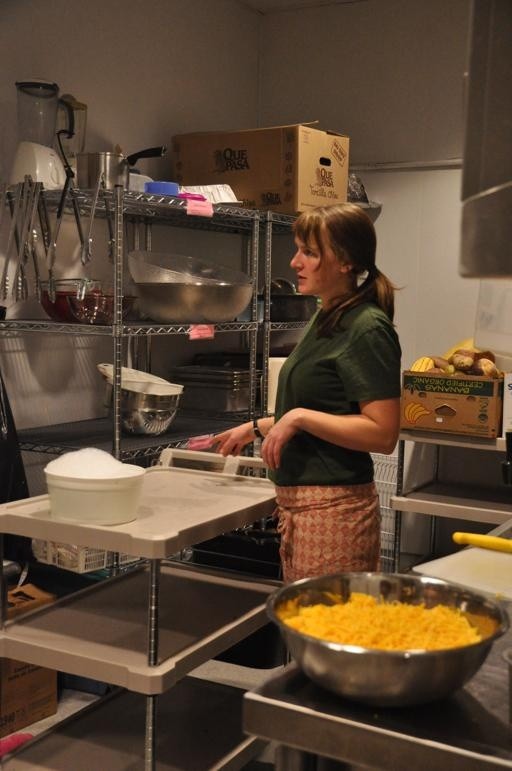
xmin=209 ymin=203 xmax=402 ymax=586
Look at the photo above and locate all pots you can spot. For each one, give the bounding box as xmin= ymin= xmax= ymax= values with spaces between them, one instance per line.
xmin=77 ymin=145 xmax=169 ymax=189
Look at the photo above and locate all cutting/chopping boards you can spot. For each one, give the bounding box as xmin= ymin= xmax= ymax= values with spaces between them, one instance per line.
xmin=412 ymin=546 xmax=512 ymax=603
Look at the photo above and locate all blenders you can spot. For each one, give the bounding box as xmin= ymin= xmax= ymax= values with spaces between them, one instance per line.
xmin=12 ymin=78 xmax=86 ymax=190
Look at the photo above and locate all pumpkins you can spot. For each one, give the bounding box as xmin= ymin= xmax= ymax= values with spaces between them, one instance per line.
xmin=410 ymin=356 xmax=434 ymax=372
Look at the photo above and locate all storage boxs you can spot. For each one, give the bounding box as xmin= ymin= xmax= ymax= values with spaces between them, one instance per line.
xmin=169 ymin=116 xmax=351 ymax=222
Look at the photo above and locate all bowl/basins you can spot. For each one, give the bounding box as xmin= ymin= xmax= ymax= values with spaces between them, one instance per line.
xmin=100 ymin=364 xmax=183 ymax=436
xmin=269 ymin=293 xmax=317 ymax=321
xmin=42 ymin=463 xmax=147 ymax=524
xmin=39 ymin=290 xmax=139 ymax=325
xmin=125 ymin=248 xmax=254 ymax=324
xmin=265 ymin=569 xmax=509 ymax=709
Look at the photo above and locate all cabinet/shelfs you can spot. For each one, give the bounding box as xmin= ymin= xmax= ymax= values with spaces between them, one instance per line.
xmin=4 ymin=186 xmax=512 ymax=769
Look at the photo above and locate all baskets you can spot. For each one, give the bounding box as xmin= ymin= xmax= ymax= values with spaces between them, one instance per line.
xmin=30 ymin=536 xmax=141 ymax=575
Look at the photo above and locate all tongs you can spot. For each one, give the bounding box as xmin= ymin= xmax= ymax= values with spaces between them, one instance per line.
xmin=0 ymin=170 xmax=116 ymax=302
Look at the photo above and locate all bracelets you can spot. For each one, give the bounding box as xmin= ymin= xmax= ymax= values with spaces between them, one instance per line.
xmin=253 ymin=417 xmax=263 ymax=437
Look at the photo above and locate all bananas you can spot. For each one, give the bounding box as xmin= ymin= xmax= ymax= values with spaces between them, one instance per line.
xmin=404 ymin=401 xmax=431 ymax=424
